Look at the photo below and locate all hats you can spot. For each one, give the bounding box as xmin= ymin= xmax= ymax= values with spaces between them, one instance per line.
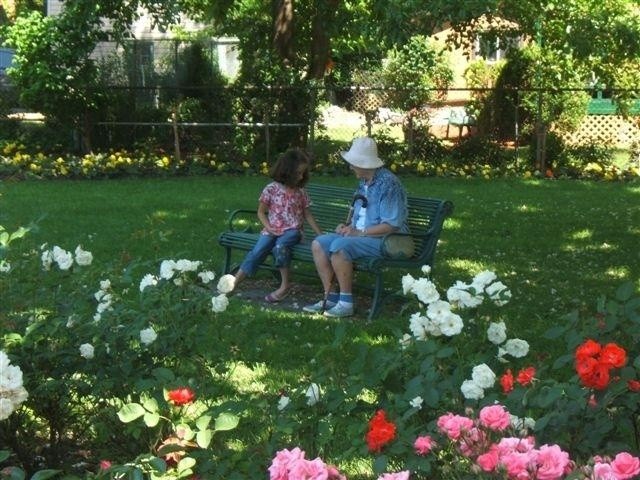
xmin=340 ymin=136 xmax=384 ymax=169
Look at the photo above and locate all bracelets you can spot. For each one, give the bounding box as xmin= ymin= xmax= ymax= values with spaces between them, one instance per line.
xmin=361 ymin=228 xmax=368 ymax=236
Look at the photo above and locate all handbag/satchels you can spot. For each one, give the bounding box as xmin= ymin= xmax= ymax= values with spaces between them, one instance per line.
xmin=382 ymin=234 xmax=416 ymax=261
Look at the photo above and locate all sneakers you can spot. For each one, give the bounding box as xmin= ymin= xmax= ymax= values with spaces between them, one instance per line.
xmin=302 ymin=300 xmax=355 ymax=317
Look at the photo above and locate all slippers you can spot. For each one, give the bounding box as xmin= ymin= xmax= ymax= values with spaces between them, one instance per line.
xmin=264 ymin=288 xmax=291 ymax=304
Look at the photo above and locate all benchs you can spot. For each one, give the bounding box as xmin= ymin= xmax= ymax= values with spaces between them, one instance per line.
xmin=218 ymin=183 xmax=456 ymax=323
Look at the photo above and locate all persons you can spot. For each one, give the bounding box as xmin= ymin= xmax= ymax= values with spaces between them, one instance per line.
xmin=226 ymin=150 xmax=322 ymax=302
xmin=303 ymin=138 xmax=410 ymax=317
